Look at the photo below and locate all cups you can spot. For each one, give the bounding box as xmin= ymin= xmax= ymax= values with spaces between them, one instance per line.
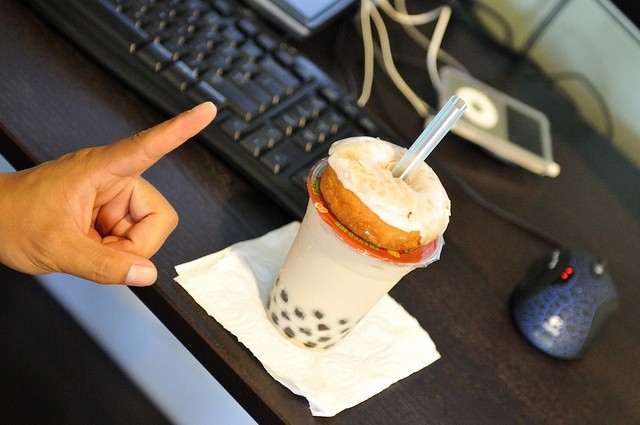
xmin=264 ymin=154 xmax=444 ymax=354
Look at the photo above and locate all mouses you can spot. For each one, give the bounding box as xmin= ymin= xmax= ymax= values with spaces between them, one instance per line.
xmin=507 ymin=245 xmax=626 ymax=365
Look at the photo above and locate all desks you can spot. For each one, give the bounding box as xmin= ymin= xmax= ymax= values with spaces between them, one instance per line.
xmin=1 ymin=0 xmax=640 ymax=423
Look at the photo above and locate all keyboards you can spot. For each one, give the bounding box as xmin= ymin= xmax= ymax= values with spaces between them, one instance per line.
xmin=28 ymin=1 xmax=411 ymax=222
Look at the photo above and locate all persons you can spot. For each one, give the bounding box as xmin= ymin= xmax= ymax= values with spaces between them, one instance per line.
xmin=1 ymin=101 xmax=218 ymax=286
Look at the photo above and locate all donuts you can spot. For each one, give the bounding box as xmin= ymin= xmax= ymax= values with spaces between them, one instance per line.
xmin=318 ymin=135 xmax=453 ymax=250
